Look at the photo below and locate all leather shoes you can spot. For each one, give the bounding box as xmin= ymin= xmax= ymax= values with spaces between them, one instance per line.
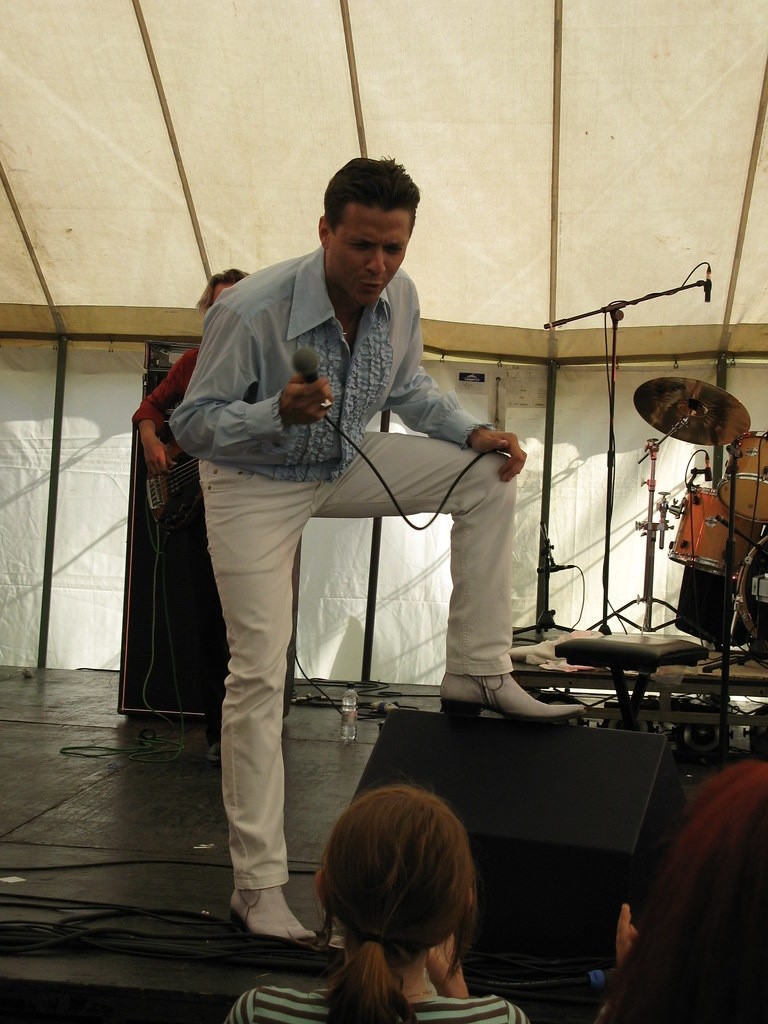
xmin=439 ymin=673 xmax=587 ymax=720
xmin=229 ymin=887 xmax=318 ymax=939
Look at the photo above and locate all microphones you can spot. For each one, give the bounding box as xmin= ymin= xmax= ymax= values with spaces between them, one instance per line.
xmin=705 ymin=454 xmax=712 ymax=481
xmin=704 ymin=265 xmax=712 ymax=303
xmin=536 ymin=564 xmax=575 ymax=574
xmin=291 ymin=347 xmax=318 ymax=384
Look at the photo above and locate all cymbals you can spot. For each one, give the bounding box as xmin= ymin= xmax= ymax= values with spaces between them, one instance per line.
xmin=632 ymin=374 xmax=752 ymax=446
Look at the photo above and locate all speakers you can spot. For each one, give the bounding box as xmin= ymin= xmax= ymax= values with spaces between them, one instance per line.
xmin=351 ymin=707 xmax=689 ymax=957
xmin=118 ymin=418 xmax=303 ymax=723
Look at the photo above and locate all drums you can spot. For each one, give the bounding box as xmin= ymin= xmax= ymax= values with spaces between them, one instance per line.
xmin=667 ymin=485 xmax=765 ymax=580
xmin=734 ymin=536 xmax=768 ymax=647
xmin=674 ymin=559 xmax=755 ymax=645
xmin=716 ymin=429 xmax=768 ymax=524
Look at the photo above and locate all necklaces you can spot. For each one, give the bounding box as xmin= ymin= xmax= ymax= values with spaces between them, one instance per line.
xmin=332 ymin=296 xmax=360 ymax=345
xmin=408 ymin=989 xmax=432 ymax=997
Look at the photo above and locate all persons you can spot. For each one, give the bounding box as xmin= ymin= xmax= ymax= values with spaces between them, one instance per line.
xmin=167 ymin=156 xmax=585 ymax=942
xmin=597 ymin=759 xmax=768 ymax=1024
xmin=132 ymin=269 xmax=250 ymax=762
xmin=219 ymin=781 xmax=529 ymax=1024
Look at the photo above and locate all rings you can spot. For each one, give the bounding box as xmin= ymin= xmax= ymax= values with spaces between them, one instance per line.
xmin=322 ymin=398 xmax=332 ymax=410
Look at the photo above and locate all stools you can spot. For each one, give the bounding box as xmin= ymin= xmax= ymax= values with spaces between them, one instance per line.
xmin=554 ymin=630 xmax=710 ymax=728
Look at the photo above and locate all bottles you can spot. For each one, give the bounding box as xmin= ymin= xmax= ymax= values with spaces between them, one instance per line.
xmin=341 ymin=682 xmax=358 ymax=741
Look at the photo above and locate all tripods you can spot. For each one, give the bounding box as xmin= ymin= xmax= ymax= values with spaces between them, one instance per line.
xmin=513 ymin=280 xmax=705 ymax=644
